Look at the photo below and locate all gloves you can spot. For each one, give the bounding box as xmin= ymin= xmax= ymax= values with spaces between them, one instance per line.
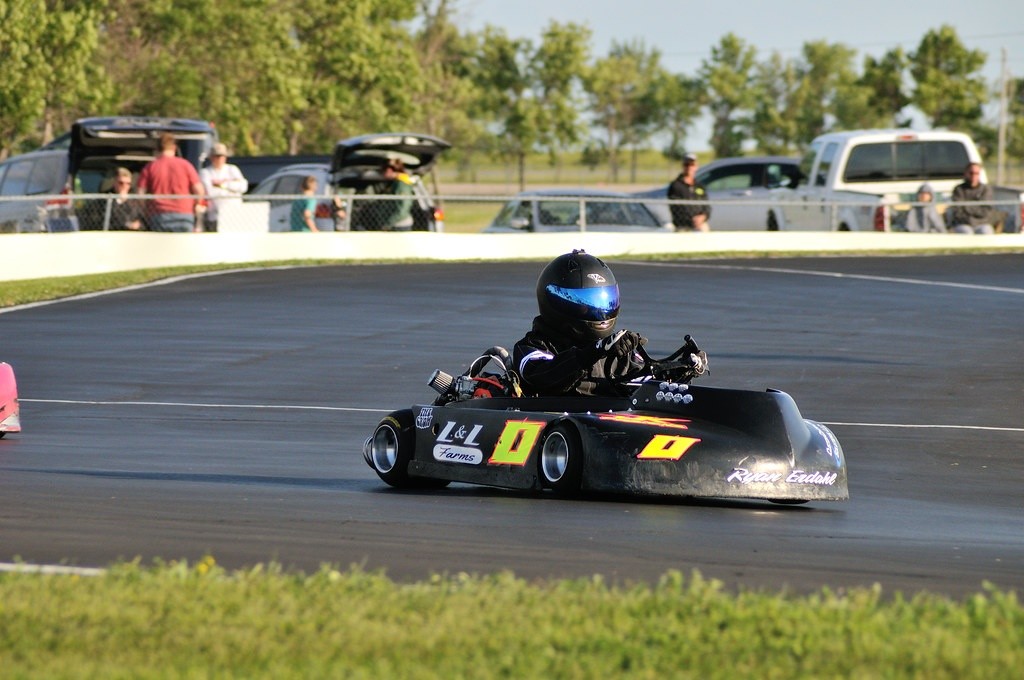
xmin=666 ymin=350 xmax=709 ymax=383
xmin=584 ymin=332 xmax=638 ymax=363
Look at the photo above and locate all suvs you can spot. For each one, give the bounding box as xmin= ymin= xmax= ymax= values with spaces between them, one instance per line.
xmin=246 ymin=132 xmax=453 ymax=233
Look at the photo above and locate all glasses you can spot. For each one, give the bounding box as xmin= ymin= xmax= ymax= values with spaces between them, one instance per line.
xmin=116 ymin=179 xmax=132 ymax=186
xmin=684 ymin=163 xmax=694 ymax=167
xmin=970 ymin=171 xmax=979 ymax=176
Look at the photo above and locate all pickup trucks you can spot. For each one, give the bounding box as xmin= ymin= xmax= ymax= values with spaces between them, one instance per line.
xmin=767 ymin=128 xmax=1024 ymax=235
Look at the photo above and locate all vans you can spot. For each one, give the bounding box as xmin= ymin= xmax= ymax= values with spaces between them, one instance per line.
xmin=0 ymin=116 xmax=219 ymax=233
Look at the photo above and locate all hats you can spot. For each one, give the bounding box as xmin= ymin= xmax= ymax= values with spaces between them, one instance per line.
xmin=684 ymin=152 xmax=697 ymax=161
xmin=210 ymin=143 xmax=228 ymax=156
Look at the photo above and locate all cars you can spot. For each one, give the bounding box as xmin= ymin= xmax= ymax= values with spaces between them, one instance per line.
xmin=631 ymin=156 xmax=802 ymax=231
xmin=479 ymin=189 xmax=667 ymax=232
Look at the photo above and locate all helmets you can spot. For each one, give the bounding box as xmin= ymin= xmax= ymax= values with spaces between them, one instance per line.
xmin=537 ymin=250 xmax=622 ymax=345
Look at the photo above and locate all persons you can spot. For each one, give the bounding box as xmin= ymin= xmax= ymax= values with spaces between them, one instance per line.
xmin=136 ymin=133 xmax=207 ymax=233
xmin=667 ymin=153 xmax=711 ymax=233
xmin=199 ymin=142 xmax=248 ymax=233
xmin=905 ymin=184 xmax=948 ymax=233
xmin=377 ymin=158 xmax=413 ymax=231
xmin=289 ymin=176 xmax=323 ymax=233
xmin=950 ymin=162 xmax=995 ymax=234
xmin=89 ymin=166 xmax=149 ymax=232
xmin=513 ymin=249 xmax=708 ymax=395
